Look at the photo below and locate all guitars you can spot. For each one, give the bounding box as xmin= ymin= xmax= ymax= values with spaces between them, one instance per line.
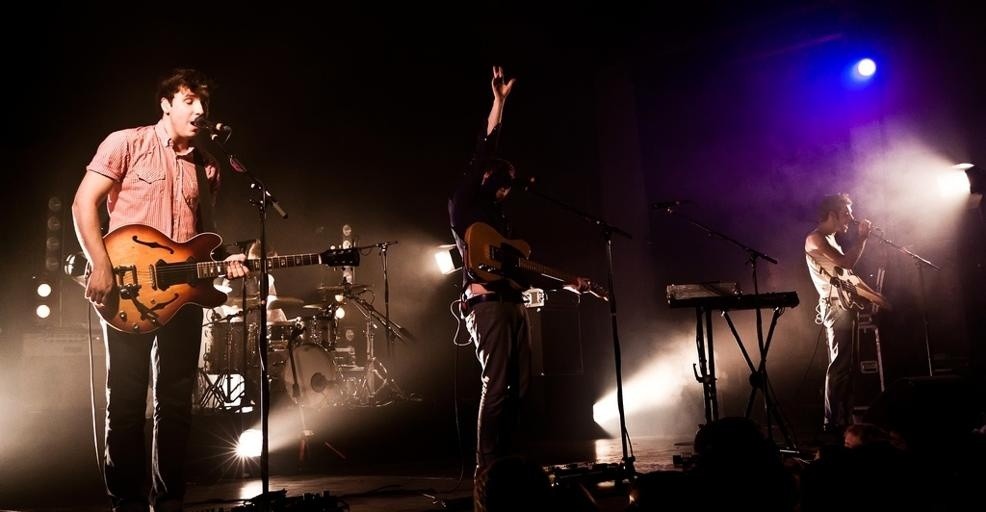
xmin=830 ymin=266 xmax=891 ymax=324
xmin=84 ymin=223 xmax=359 ymax=336
xmin=464 ymin=222 xmax=610 ymax=303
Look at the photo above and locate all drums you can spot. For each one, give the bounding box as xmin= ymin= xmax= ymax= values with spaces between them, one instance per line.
xmin=301 ymin=315 xmax=338 ymax=352
xmin=248 ymin=322 xmax=304 ymax=381
xmin=203 ymin=321 xmax=257 ymax=373
xmin=267 ymin=342 xmax=338 ymax=410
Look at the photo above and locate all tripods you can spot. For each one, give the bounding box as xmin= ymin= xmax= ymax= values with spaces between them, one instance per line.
xmin=278 ymin=346 xmax=351 ymax=471
xmin=350 ymin=319 xmax=405 ymax=403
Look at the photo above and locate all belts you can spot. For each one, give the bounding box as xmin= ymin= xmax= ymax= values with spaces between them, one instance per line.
xmin=469 ymin=293 xmax=522 ymax=306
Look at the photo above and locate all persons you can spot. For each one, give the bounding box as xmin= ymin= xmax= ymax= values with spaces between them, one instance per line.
xmin=445 ymin=64 xmax=592 ymax=512
xmin=207 ymin=240 xmax=304 ymax=407
xmin=803 ymin=191 xmax=872 ymax=456
xmin=70 ymin=69 xmax=224 ymax=512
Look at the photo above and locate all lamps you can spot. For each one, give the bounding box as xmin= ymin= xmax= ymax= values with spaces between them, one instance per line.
xmin=434 ymin=242 xmax=463 ymax=276
xmin=951 ymin=162 xmax=986 ymax=198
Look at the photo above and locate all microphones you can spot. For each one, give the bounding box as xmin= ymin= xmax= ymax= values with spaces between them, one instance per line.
xmin=652 ymin=198 xmax=682 ymax=208
xmin=851 ymin=217 xmax=878 ymax=230
xmin=519 ymin=183 xmax=530 ymax=193
xmin=195 ymin=115 xmax=228 ymax=136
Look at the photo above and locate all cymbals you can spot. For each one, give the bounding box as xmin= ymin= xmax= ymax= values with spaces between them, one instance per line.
xmin=302 ymin=301 xmax=346 ymax=310
xmin=236 ymin=295 xmax=306 ymax=310
xmin=317 ymin=282 xmax=372 ymax=292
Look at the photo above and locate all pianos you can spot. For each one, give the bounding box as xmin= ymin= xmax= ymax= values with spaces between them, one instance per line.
xmin=666 ymin=282 xmax=799 ymax=310
